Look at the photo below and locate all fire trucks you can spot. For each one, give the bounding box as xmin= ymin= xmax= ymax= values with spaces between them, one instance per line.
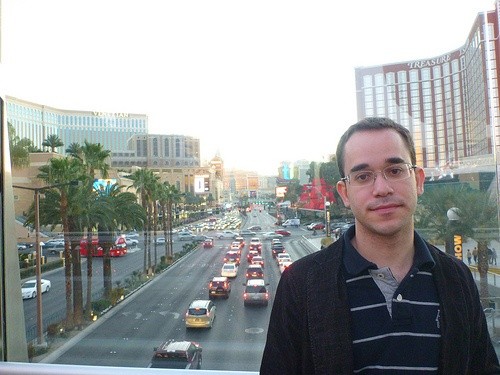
xmin=80 ymin=232 xmax=128 ymax=256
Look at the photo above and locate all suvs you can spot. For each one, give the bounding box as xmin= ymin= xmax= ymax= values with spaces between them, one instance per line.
xmin=242 ymin=278 xmax=269 ymax=305
xmin=185 ymin=299 xmax=216 ymax=329
xmin=207 ymin=275 xmax=231 ymax=298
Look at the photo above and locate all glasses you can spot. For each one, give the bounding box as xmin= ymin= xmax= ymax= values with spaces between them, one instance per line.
xmin=341 ymin=164 xmax=418 ymax=187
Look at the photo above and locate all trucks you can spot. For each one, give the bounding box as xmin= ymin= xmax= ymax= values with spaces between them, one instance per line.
xmin=281 ymin=218 xmax=301 ymax=227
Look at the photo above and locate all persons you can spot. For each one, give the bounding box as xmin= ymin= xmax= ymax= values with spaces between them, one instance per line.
xmin=260 ymin=118 xmax=500 ymax=375
xmin=467 ymin=247 xmax=497 ymax=265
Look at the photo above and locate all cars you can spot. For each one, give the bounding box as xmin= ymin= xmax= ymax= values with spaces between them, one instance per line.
xmin=307 ymin=220 xmax=355 ymax=235
xmin=151 ymin=238 xmax=175 ymax=245
xmin=224 ymin=226 xmax=295 ymax=272
xmin=245 ymin=263 xmax=265 ymax=280
xmin=173 ymin=213 xmax=246 ymax=247
xmin=127 ymin=231 xmax=141 ymax=245
xmin=17 ymin=239 xmax=69 ymax=257
xmin=18 ymin=279 xmax=51 ymax=300
xmin=221 ymin=263 xmax=237 ymax=279
xmin=151 ymin=337 xmax=205 ymax=369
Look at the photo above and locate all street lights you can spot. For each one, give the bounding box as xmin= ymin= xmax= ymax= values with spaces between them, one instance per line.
xmin=13 ymin=180 xmax=77 ymax=351
xmin=444 ymin=207 xmax=464 ymax=259
xmin=325 ymin=202 xmax=332 ymax=236
